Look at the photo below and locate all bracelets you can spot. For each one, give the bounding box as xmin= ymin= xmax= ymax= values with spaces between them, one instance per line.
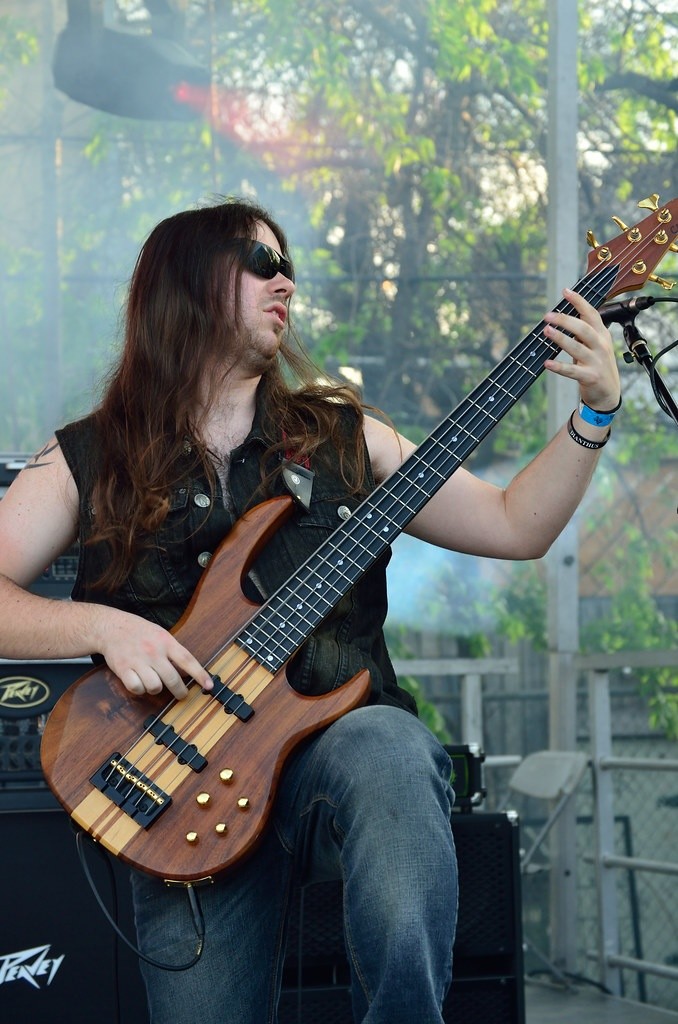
xmin=569 ymin=395 xmax=622 ymax=449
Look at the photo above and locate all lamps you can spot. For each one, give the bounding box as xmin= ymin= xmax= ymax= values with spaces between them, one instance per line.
xmin=53 ymin=0 xmax=213 ymax=118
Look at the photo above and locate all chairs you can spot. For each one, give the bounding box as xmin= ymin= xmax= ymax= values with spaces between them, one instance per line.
xmin=502 ymin=751 xmax=590 ymax=994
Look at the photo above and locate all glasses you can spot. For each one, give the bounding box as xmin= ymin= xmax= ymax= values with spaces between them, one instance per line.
xmin=229 ymin=238 xmax=295 ymax=284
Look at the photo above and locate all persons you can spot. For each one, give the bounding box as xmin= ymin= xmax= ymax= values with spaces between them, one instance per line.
xmin=0 ymin=201 xmax=622 ymax=1024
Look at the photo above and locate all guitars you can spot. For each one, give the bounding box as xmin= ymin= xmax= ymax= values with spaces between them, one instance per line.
xmin=37 ymin=192 xmax=678 ymax=883
